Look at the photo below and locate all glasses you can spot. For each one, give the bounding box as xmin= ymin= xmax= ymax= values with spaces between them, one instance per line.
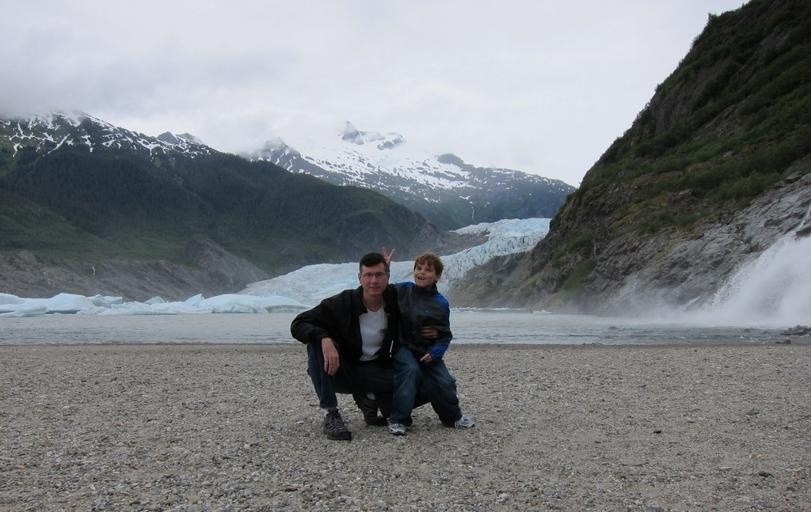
xmin=362 ymin=272 xmax=386 ymax=280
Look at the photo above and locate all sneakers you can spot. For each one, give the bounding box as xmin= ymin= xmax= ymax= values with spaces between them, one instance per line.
xmin=455 ymin=415 xmax=474 ymax=429
xmin=388 ymin=420 xmax=407 ymax=435
xmin=353 ymin=392 xmax=387 ymax=426
xmin=323 ymin=410 xmax=351 ymax=440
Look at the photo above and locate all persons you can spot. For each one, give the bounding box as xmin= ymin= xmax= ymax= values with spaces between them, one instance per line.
xmin=381 ymin=245 xmax=474 ymax=435
xmin=290 ymin=254 xmax=441 ymax=441
